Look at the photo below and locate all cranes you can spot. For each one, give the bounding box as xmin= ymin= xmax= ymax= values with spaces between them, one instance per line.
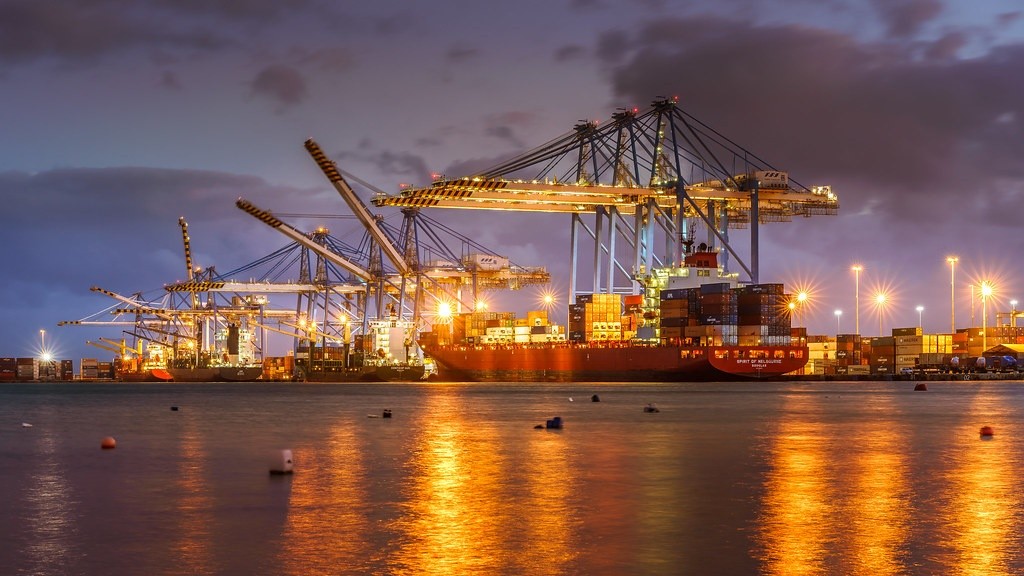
xmin=52 ymin=92 xmax=844 ymax=370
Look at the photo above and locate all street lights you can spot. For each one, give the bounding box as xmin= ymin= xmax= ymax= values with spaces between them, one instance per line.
xmin=981 ymin=285 xmax=992 ymax=352
xmin=798 ymin=293 xmax=807 ymax=328
xmin=969 ymin=284 xmax=975 ymax=328
xmin=948 ymin=257 xmax=959 ymax=334
xmin=917 ymin=306 xmax=924 ymax=327
xmin=877 ymin=294 xmax=886 ymax=336
xmin=40 ymin=329 xmax=45 ymax=362
xmin=852 ymin=266 xmax=863 ymax=334
xmin=834 ymin=310 xmax=842 ymax=333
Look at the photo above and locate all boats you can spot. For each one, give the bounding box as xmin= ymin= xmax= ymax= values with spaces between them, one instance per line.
xmin=295 ymin=365 xmax=425 ymax=382
xmin=165 ymin=367 xmax=264 ymax=382
xmin=413 ymin=337 xmax=809 ymax=381
xmin=114 ymin=368 xmax=172 ymax=382
